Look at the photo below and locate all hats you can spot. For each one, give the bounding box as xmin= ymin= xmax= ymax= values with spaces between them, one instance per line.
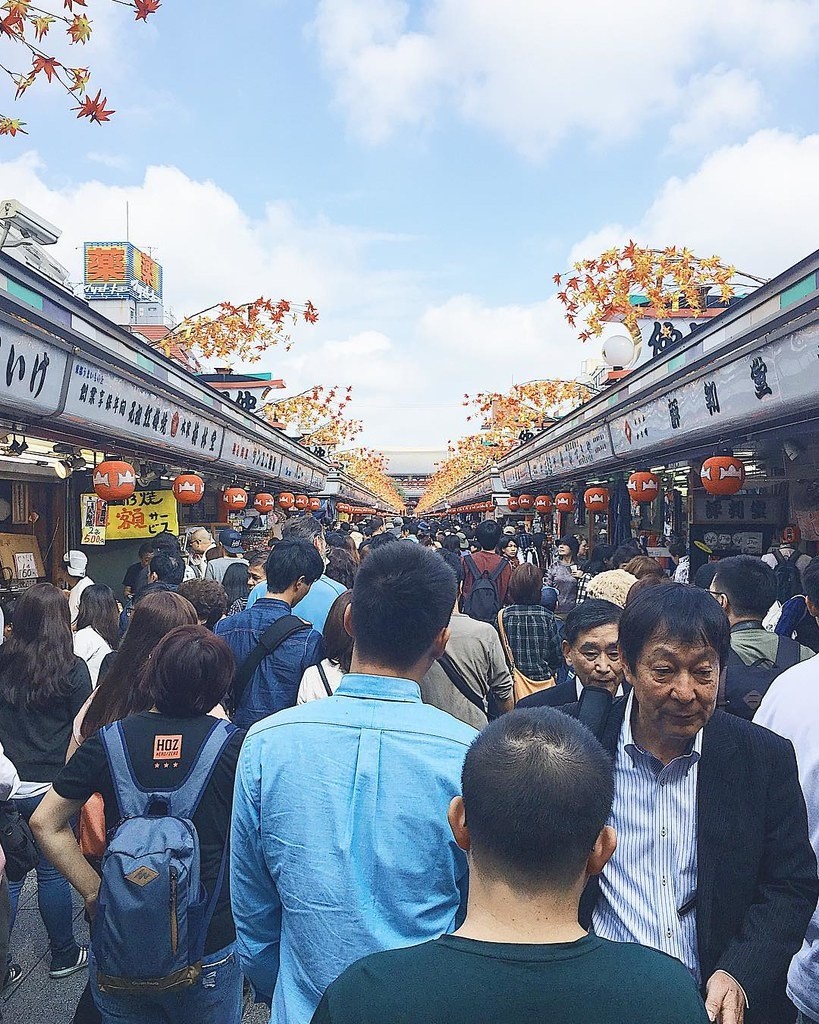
xmin=780 ymin=524 xmax=801 ymax=544
xmin=555 ymin=534 xmax=579 ymax=558
xmin=62 ymin=550 xmax=87 ymax=577
xmin=417 ymin=522 xmax=430 ymax=530
xmin=219 ymin=530 xmax=243 ymax=554
xmin=504 ymin=526 xmax=515 ymax=536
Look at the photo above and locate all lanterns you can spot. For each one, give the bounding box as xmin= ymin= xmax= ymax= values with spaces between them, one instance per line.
xmin=92 ymin=457 xmax=136 ymax=505
xmin=517 ymin=493 xmax=534 ymax=511
xmin=628 ymin=468 xmax=660 ymax=506
xmin=439 ymin=501 xmax=495 ymax=518
xmin=701 ymin=452 xmax=745 ymax=501
xmin=171 ymin=471 xmax=204 ymax=508
xmin=534 ymin=494 xmax=552 ymax=514
xmin=309 ymin=498 xmax=320 ymax=510
xmin=223 ymin=488 xmax=247 ymax=513
xmin=583 ymin=484 xmax=609 ymax=514
xmin=555 ymin=490 xmax=575 ymax=514
xmin=294 ymin=496 xmax=308 ymax=510
xmin=507 ymin=495 xmax=520 ymax=512
xmin=253 ymin=493 xmax=274 ymax=515
xmin=335 ymin=503 xmax=388 ymax=516
xmin=277 ymin=493 xmax=295 ymax=509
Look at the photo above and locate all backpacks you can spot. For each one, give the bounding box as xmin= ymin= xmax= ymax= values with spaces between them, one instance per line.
xmin=89 ymin=721 xmax=239 ymax=998
xmin=718 ymin=633 xmax=802 ymax=722
xmin=772 ymin=550 xmax=802 ymax=604
xmin=462 ymin=555 xmax=508 ymax=622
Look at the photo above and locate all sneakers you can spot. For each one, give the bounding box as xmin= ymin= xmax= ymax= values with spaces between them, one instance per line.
xmin=48 ymin=943 xmax=89 ymax=977
xmin=3 ymin=963 xmax=21 ymax=987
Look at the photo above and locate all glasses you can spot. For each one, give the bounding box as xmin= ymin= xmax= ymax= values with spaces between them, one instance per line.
xmin=188 ymin=539 xmax=201 ymax=547
xmin=705 ymin=588 xmax=730 ymax=607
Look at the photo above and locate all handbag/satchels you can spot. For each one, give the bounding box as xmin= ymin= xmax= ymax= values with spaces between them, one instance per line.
xmin=761 ymin=595 xmax=807 ymax=640
xmin=512 ymin=666 xmax=560 ymax=703
xmin=0 ymin=798 xmax=42 ymax=883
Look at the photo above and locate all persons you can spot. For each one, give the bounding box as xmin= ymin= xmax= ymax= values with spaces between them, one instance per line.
xmin=0 ymin=514 xmax=819 ymax=1024
